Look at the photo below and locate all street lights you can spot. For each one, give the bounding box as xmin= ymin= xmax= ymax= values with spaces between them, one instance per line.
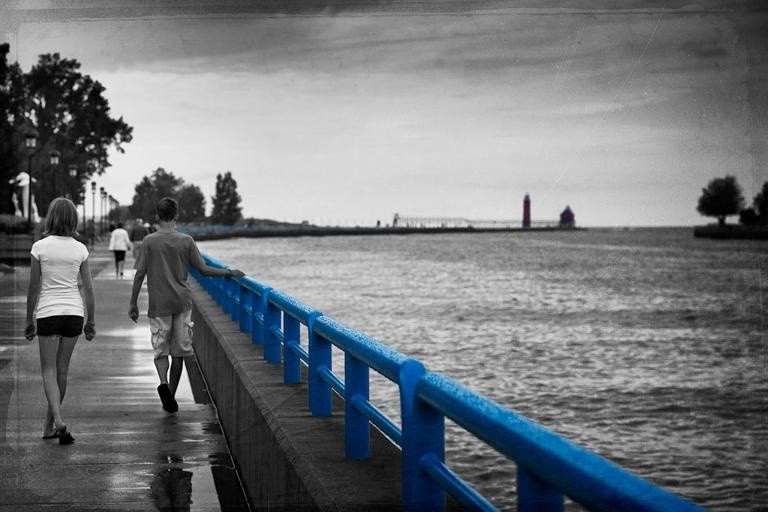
xmin=70 ymin=162 xmax=120 ymax=249
xmin=49 ymin=149 xmax=61 ymax=198
xmin=24 ymin=125 xmax=39 ymax=236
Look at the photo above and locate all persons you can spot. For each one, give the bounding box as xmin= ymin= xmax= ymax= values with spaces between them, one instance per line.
xmin=24 ymin=196 xmax=97 ymax=447
xmin=109 ymin=217 xmax=160 ymax=280
xmin=127 ymin=197 xmax=245 ymax=414
xmin=150 ymin=455 xmax=193 ymax=512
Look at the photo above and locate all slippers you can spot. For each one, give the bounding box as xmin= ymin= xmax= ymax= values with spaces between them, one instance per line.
xmin=41 ymin=428 xmax=61 ymax=440
xmin=57 ymin=427 xmax=75 ymax=444
xmin=157 ymin=383 xmax=178 ymax=412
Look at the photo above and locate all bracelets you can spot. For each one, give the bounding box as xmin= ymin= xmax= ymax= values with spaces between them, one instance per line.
xmin=228 ymin=269 xmax=233 ymax=276
xmin=86 ymin=321 xmax=95 ymax=327
